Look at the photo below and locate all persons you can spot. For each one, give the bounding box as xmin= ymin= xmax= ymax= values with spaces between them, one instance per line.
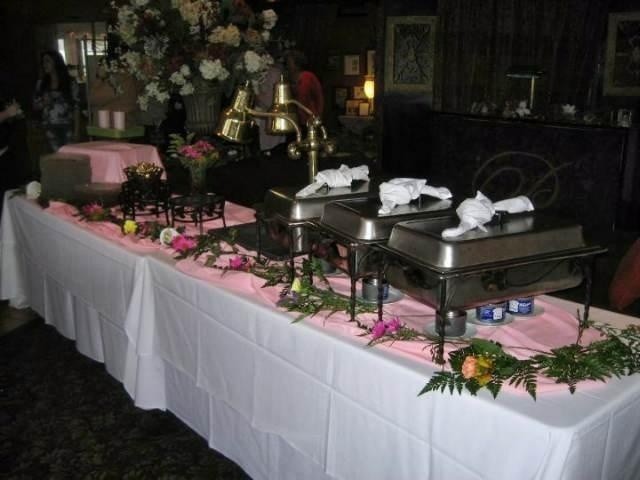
xmin=288 ymin=50 xmax=324 ymax=188
xmin=159 ymin=93 xmax=192 ymax=143
xmin=33 ymin=50 xmax=80 ymax=153
xmin=0 ymin=46 xmax=33 ymax=211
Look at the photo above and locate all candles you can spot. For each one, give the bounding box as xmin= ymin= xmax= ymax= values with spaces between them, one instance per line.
xmin=113 ymin=112 xmax=126 ymax=128
xmin=97 ymin=110 xmax=110 ymax=128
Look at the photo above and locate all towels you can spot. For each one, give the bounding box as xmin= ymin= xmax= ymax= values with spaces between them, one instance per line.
xmin=441 ymin=191 xmax=536 ymax=241
xmin=296 ymin=163 xmax=370 ymax=199
xmin=377 ymin=177 xmax=453 ymax=217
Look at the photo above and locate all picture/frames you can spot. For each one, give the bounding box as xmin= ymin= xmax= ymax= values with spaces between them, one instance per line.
xmin=385 ymin=15 xmax=441 ymax=95
xmin=603 ymin=12 xmax=640 ymax=96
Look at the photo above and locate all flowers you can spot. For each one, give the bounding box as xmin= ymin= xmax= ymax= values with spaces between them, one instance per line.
xmin=274 ymin=256 xmax=380 ymax=327
xmin=120 ymin=216 xmax=186 ymax=246
xmin=213 ymin=253 xmax=301 ymax=288
xmin=167 ymin=126 xmax=220 ymax=171
xmin=8 ymin=180 xmax=50 ymax=209
xmin=72 ymin=196 xmax=123 ymax=227
xmin=414 ymin=308 xmax=640 ymax=402
xmin=164 ymin=228 xmax=259 ymax=261
xmin=95 ymin=0 xmax=298 ymax=113
xmin=353 ymin=315 xmax=555 ymax=356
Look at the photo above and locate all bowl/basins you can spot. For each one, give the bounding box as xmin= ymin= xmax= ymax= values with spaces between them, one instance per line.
xmin=322 ymin=197 xmax=459 ymax=252
xmin=384 ymin=217 xmax=587 ymax=308
xmin=262 ymin=183 xmax=380 ymax=251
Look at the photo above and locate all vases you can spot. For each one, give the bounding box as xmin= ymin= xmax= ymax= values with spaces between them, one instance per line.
xmin=190 ymin=171 xmax=209 ymax=207
xmin=173 ymin=89 xmax=221 ymax=135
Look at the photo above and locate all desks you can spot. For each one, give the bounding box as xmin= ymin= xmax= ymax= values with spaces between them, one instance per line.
xmin=0 ymin=189 xmax=640 ymax=480
xmin=430 ymin=114 xmax=639 ymax=241
xmin=56 ymin=140 xmax=167 ymax=188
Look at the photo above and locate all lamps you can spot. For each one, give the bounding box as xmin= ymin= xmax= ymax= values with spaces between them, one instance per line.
xmin=214 ymin=74 xmax=337 ymax=185
xmin=505 ymin=66 xmax=541 ymax=118
xmin=363 ymin=80 xmax=374 ymax=99
